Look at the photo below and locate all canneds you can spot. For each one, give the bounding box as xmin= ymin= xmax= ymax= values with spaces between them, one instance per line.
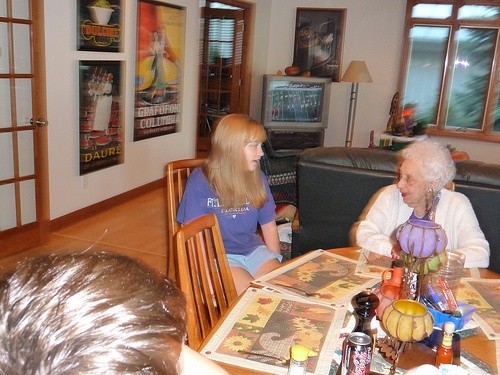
xmin=341 ymin=332 xmax=372 ymax=375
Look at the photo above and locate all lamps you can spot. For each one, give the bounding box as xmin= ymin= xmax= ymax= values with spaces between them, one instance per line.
xmin=340 ymin=59 xmax=373 ymax=147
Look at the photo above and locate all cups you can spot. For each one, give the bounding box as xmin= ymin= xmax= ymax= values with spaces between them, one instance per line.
xmin=429 ymin=248 xmax=466 ymax=300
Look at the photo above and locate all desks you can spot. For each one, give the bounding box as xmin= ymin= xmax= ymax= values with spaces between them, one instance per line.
xmin=196 ymin=246 xmax=500 ymax=375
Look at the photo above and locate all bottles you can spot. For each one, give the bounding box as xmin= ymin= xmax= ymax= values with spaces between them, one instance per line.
xmin=436 ymin=321 xmax=455 ymax=368
xmin=287 ymin=344 xmax=309 ymax=375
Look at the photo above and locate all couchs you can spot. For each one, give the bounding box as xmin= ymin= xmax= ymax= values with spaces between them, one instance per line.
xmin=292 ymin=145 xmax=500 ymax=271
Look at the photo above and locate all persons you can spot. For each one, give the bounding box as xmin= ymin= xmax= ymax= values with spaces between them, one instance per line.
xmin=88 ymin=67 xmax=113 ymax=133
xmin=145 ymin=32 xmax=169 ymax=99
xmin=0 ymin=249 xmax=230 ymax=375
xmin=348 ymin=140 xmax=490 ymax=269
xmin=176 ymin=113 xmax=282 ymax=296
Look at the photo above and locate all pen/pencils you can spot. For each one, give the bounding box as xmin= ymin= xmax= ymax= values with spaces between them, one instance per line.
xmin=422 ymin=276 xmax=458 ymax=313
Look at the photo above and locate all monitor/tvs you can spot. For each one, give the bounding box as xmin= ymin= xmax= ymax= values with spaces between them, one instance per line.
xmin=261 ymin=73 xmax=331 ymax=133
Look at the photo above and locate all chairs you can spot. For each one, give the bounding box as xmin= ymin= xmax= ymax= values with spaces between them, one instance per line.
xmin=164 ymin=158 xmax=238 ymax=345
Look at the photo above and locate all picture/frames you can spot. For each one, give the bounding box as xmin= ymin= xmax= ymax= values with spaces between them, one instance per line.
xmin=293 ymin=7 xmax=348 ymax=83
